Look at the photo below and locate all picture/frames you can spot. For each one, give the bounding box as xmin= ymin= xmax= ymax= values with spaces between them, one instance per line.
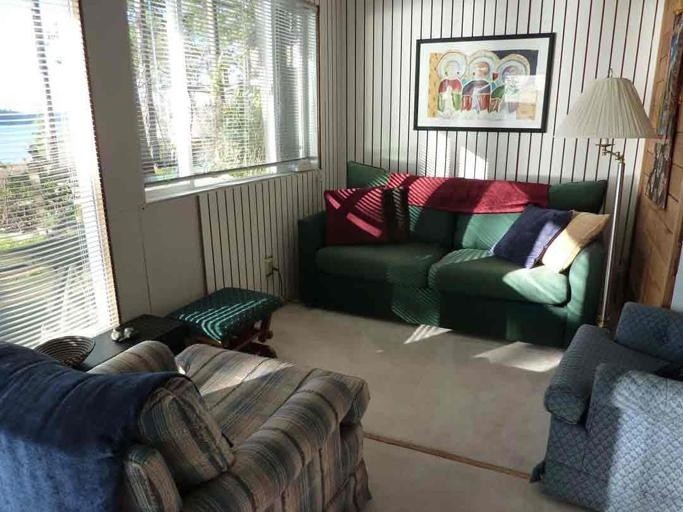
xmin=411 ymin=31 xmax=555 ymax=132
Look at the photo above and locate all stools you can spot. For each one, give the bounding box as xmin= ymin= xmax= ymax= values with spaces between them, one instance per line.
xmin=170 ymin=285 xmax=284 ymax=342
xmin=537 ymin=300 xmax=683 ymax=512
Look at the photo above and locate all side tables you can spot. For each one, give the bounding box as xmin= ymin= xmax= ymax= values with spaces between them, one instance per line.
xmin=70 ymin=314 xmax=203 ymax=372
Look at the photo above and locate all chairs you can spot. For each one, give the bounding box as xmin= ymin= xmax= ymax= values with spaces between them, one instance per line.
xmin=1 ymin=335 xmax=373 ymax=512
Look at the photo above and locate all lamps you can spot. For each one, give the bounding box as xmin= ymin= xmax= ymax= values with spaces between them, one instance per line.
xmin=553 ymin=64 xmax=659 ymax=325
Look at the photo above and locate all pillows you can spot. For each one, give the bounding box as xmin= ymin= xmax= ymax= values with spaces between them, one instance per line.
xmin=322 ymin=183 xmax=393 ymax=246
xmin=490 ymin=203 xmax=609 ymax=273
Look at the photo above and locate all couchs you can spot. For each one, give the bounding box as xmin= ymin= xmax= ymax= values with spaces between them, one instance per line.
xmin=296 ymin=161 xmax=609 ymax=346
